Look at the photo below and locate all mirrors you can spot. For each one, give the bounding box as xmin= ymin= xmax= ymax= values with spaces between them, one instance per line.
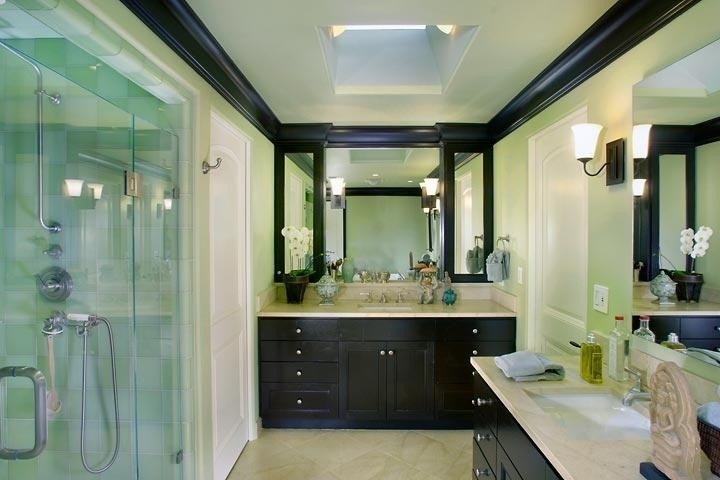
xmin=628 ymin=34 xmax=720 ymax=369
xmin=651 ymin=143 xmax=696 ymax=281
xmin=322 ymin=141 xmax=446 ymax=283
xmin=272 ymin=140 xmax=324 ymax=284
xmin=447 ymin=138 xmax=495 ymax=283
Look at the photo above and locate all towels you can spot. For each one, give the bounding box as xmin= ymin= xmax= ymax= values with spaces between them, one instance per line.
xmin=473 ymin=246 xmax=483 ymax=270
xmin=695 ymin=398 xmax=720 ymax=429
xmin=492 ymin=349 xmax=566 ymax=384
xmin=493 ymin=248 xmax=512 ymax=281
xmin=685 ymin=347 xmax=720 ymax=368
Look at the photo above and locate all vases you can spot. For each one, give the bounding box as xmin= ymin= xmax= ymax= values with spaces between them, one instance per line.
xmin=671 ymin=271 xmax=705 ymax=303
xmin=284 ymin=276 xmax=310 ymax=304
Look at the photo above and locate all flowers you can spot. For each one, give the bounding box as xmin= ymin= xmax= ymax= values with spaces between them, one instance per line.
xmin=307 ymin=230 xmax=313 ymax=271
xmin=653 ymin=225 xmax=714 ymax=275
xmin=281 ymin=224 xmax=340 ymax=276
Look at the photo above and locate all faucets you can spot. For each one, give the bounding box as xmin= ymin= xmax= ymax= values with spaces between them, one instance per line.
xmin=622 ymin=365 xmax=652 ymax=407
xmin=379 ymin=293 xmax=388 ymax=304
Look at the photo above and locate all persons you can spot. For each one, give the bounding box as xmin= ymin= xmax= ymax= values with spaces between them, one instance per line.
xmin=649 ymin=390 xmax=680 ymax=449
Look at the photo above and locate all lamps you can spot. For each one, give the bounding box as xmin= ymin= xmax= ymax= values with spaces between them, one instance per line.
xmin=63 ymin=177 xmax=85 ymax=199
xmin=632 ymin=176 xmax=648 ymax=199
xmin=156 ymin=198 xmax=172 ymax=217
xmin=327 ymin=177 xmax=347 ymax=210
xmin=571 ymin=122 xmax=627 ymax=188
xmin=633 ymin=122 xmax=653 ymax=178
xmin=87 ymin=180 xmax=104 ymax=201
xmin=418 ymin=177 xmax=441 ymax=223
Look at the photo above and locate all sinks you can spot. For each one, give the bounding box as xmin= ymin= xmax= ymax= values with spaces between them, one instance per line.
xmin=357 ymin=302 xmax=413 ymax=312
xmin=521 ymin=386 xmax=651 ymax=443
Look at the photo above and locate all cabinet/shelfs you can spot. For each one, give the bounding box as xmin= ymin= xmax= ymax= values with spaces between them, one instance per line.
xmin=470 ymin=360 xmax=562 ymax=478
xmin=633 ymin=312 xmax=720 ymax=352
xmin=257 ymin=315 xmax=339 ymax=428
xmin=435 ymin=317 xmax=516 ymax=430
xmin=338 ymin=319 xmax=437 ymax=430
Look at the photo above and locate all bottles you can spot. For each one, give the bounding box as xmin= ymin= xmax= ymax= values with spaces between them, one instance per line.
xmin=659 ymin=332 xmax=687 ymax=351
xmin=607 ymin=315 xmax=630 ymax=382
xmin=577 ymin=333 xmax=603 ymax=384
xmin=651 ymin=270 xmax=679 ymax=304
xmin=634 ymin=314 xmax=657 ymax=345
xmin=342 ymin=257 xmax=354 ymax=283
xmin=313 ymin=271 xmax=339 ymax=305
